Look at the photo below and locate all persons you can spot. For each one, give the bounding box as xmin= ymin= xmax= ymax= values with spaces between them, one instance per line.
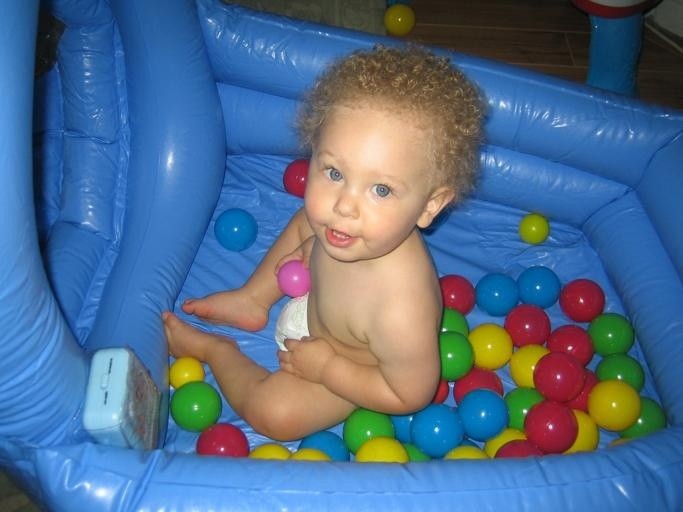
xmin=161 ymin=45 xmax=492 ymax=443
xmin=162 ymin=45 xmax=492 ymax=443
